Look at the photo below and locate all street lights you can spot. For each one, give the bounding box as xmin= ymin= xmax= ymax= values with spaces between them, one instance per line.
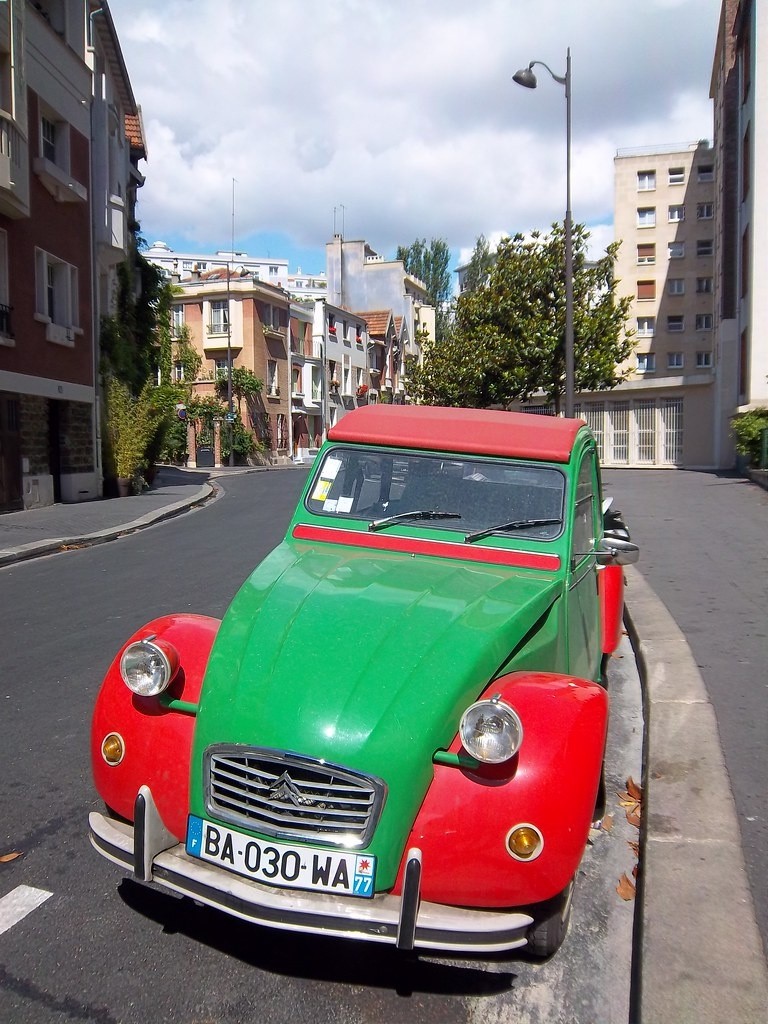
xmin=224 ymin=263 xmax=250 ymax=468
xmin=511 ymin=46 xmax=576 ymax=418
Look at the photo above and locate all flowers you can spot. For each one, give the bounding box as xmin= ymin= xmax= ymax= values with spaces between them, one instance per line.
xmin=356 ymin=385 xmax=368 ymax=396
xmin=356 ymin=335 xmax=363 ymax=343
xmin=329 ymin=326 xmax=336 ymax=334
xmin=330 ymin=380 xmax=340 ymax=386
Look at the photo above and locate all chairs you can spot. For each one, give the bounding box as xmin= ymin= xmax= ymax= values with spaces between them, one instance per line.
xmin=397 ymin=478 xmax=458 ymax=521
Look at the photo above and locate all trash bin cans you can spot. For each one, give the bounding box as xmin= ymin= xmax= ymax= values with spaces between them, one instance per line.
xmin=196 ymin=443 xmax=215 ymax=467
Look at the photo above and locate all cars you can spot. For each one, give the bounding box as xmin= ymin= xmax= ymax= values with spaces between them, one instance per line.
xmin=85 ymin=402 xmax=638 ymax=961
